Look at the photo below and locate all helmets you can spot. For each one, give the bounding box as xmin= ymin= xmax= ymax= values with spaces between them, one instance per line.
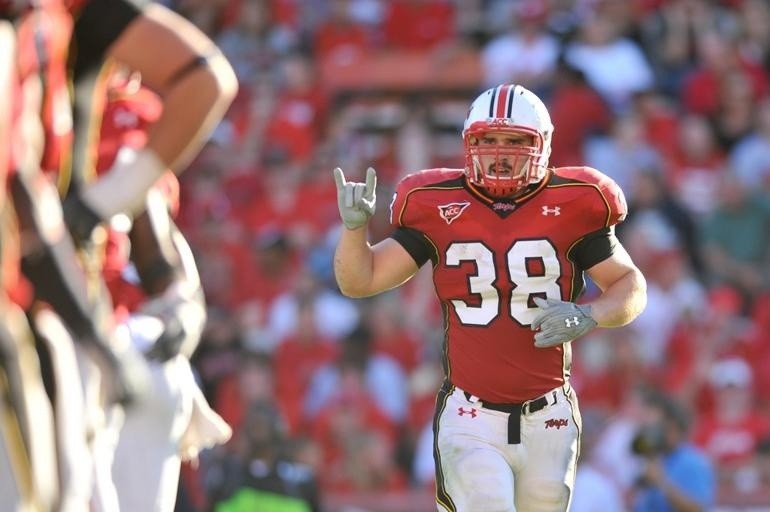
xmin=462 ymin=84 xmax=554 ymax=198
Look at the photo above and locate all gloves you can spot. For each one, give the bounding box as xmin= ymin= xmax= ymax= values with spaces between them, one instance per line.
xmin=333 ymin=167 xmax=376 ymax=230
xmin=531 ymin=298 xmax=599 ymax=348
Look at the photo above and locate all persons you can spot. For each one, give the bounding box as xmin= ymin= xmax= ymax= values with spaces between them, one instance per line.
xmin=2 ymin=1 xmax=768 ymax=510
